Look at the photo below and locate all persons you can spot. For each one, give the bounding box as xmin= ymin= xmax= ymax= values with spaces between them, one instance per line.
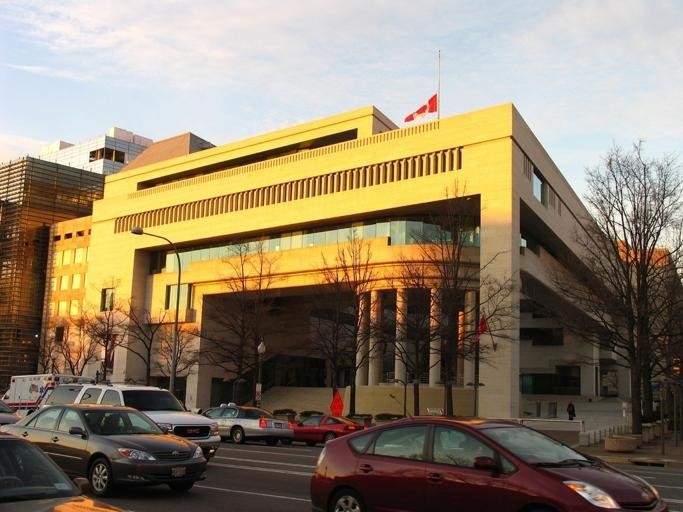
xmin=567 ymin=400 xmax=574 ymax=420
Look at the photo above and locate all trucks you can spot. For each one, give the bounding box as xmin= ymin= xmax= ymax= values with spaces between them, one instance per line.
xmin=2 ymin=375 xmax=97 ymax=409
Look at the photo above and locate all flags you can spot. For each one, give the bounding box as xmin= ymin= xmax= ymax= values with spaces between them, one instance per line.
xmin=471 ymin=315 xmax=488 ymax=347
xmin=404 ymin=94 xmax=436 ymax=123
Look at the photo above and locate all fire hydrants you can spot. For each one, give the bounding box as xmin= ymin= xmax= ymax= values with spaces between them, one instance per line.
xmin=567 ymin=402 xmax=577 ymax=420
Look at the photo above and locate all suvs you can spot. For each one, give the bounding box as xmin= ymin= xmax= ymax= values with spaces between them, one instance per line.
xmin=37 ymin=383 xmax=220 ymax=463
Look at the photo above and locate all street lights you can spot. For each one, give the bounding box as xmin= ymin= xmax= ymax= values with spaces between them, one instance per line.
xmin=130 ymin=226 xmax=182 ymax=394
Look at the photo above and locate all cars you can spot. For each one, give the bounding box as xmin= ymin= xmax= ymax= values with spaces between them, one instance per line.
xmin=1 ymin=402 xmax=207 ymax=496
xmin=309 ymin=415 xmax=666 ymax=511
xmin=291 ymin=415 xmax=367 ymax=446
xmin=206 ymin=404 xmax=294 ymax=446
xmin=1 ymin=432 xmax=120 ymax=512
